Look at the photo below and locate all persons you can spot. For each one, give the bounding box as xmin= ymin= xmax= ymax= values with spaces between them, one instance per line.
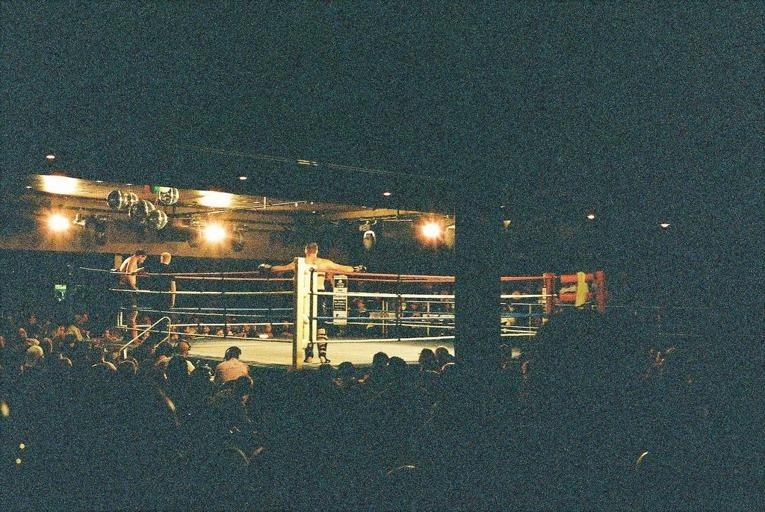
xmin=1 ymin=308 xmax=124 ymax=350
xmin=170 ymin=318 xmax=293 ymax=340
xmin=130 ymin=250 xmax=176 ymax=347
xmin=2 ymin=336 xmax=218 ymax=511
xmin=350 ymin=298 xmax=370 ymax=338
xmin=212 ymin=348 xmax=429 ymax=507
xmin=257 ymin=241 xmax=368 ymax=364
xmin=117 ymin=247 xmax=148 ymax=346
xmin=417 ymin=306 xmax=764 ymax=511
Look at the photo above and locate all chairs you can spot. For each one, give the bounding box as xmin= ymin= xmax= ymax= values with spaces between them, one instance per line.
xmin=302 ymin=352 xmax=331 ymax=362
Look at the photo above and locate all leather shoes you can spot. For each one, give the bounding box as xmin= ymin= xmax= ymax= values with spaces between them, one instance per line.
xmin=257 ymin=262 xmax=272 ymax=271
xmin=353 ymin=264 xmax=366 ymax=273
